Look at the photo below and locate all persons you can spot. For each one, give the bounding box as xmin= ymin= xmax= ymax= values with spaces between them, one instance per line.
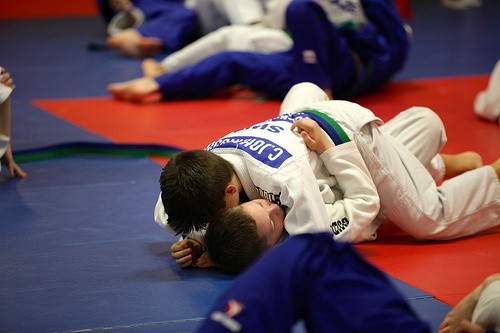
xmin=204 ymin=82 xmax=447 ymax=277
xmin=152 ymin=100 xmax=500 ymax=269
xmin=0 ymin=82 xmax=28 ymax=179
xmin=0 ymin=0 xmax=500 ymax=124
xmin=438 ymin=273 xmax=500 ymax=333
xmin=195 ymin=230 xmax=493 ymax=333
